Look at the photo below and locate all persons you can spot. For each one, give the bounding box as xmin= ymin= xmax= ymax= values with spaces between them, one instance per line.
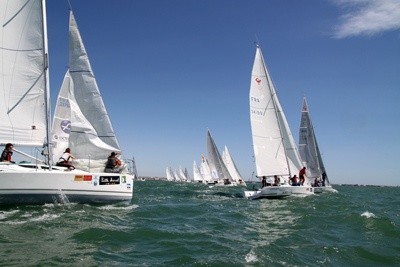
xmin=289 ymin=166 xmax=306 ymax=186
xmin=104 ymin=152 xmax=125 ymax=174
xmin=0 ymin=143 xmax=15 ymax=164
xmin=262 ymin=176 xmax=267 ymax=188
xmin=56 ymin=148 xmax=78 ymax=171
xmin=273 ymin=175 xmax=281 ymax=186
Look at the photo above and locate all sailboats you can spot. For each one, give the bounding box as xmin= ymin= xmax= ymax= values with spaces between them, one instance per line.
xmin=0 ymin=0 xmax=135 ymax=202
xmin=242 ymin=44 xmax=315 ymax=201
xmin=166 ymin=129 xmax=247 ymax=187
xmin=297 ymin=97 xmax=334 ymax=193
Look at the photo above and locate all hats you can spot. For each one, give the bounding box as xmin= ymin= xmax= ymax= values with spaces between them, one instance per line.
xmin=111 ymin=151 xmax=118 ymax=156
